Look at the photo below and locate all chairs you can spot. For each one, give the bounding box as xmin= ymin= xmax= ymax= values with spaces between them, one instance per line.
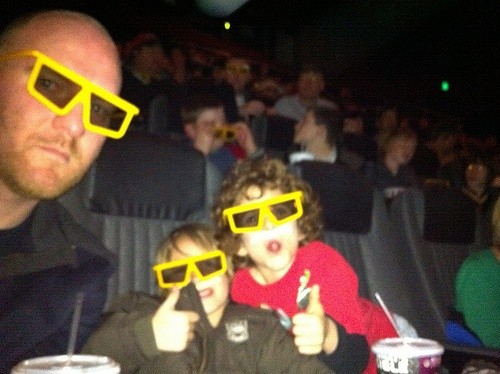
xmin=59 ymin=132 xmax=483 ymax=339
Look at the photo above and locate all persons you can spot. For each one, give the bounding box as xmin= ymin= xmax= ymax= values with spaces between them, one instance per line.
xmin=444 ymin=196 xmax=500 ymax=350
xmin=209 ymin=158 xmax=399 ymax=374
xmin=107 ymin=31 xmax=500 ymax=245
xmin=80 ymin=224 xmax=338 ymax=374
xmin=0 ymin=11 xmax=140 ymax=374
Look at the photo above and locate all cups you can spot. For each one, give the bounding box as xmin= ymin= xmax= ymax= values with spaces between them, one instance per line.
xmin=11 ymin=354 xmax=121 ymax=374
xmin=370 ymin=338 xmax=445 ymax=374
